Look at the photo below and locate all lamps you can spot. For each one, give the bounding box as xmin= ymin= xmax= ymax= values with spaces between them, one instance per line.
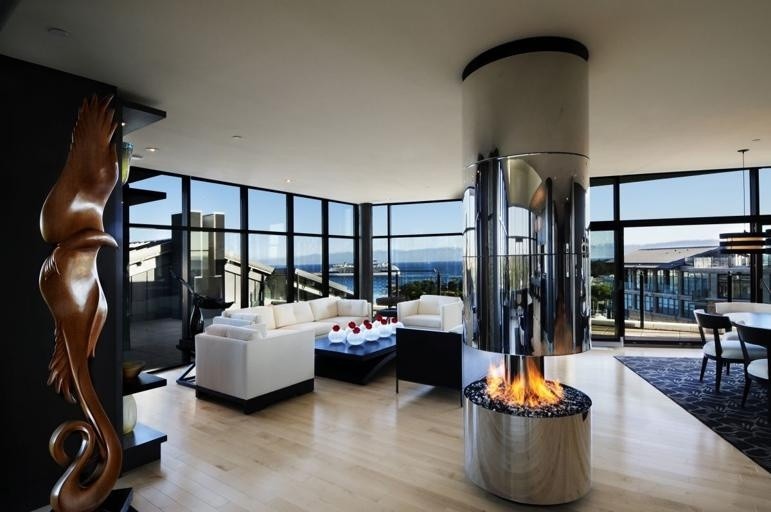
xmin=720 ymin=150 xmax=770 ymax=254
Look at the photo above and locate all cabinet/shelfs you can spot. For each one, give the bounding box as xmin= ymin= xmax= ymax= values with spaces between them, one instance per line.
xmin=118 ymin=88 xmax=167 ymax=477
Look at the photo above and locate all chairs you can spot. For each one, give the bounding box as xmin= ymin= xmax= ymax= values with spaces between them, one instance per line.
xmin=194 ymin=317 xmax=315 ymax=415
xmin=693 ymin=302 xmax=771 ymax=415
xmin=396 ymin=324 xmax=462 ymax=408
xmin=397 ymin=294 xmax=464 ymax=332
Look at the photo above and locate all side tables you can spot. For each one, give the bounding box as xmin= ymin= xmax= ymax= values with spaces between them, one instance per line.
xmin=176 ymin=339 xmax=197 ymax=389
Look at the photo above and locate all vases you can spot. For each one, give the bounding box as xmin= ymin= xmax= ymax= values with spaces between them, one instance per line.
xmin=190 ymin=293 xmax=206 ymax=332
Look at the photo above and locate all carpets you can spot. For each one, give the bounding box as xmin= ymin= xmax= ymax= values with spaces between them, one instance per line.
xmin=613 ymin=354 xmax=771 ymax=475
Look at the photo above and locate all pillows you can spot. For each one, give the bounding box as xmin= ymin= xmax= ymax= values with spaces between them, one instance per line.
xmin=206 ymin=317 xmax=267 ymax=341
xmin=225 ymin=297 xmax=368 ymax=329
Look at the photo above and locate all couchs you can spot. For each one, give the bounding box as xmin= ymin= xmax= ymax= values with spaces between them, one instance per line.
xmin=221 ymin=296 xmax=372 ymax=340
